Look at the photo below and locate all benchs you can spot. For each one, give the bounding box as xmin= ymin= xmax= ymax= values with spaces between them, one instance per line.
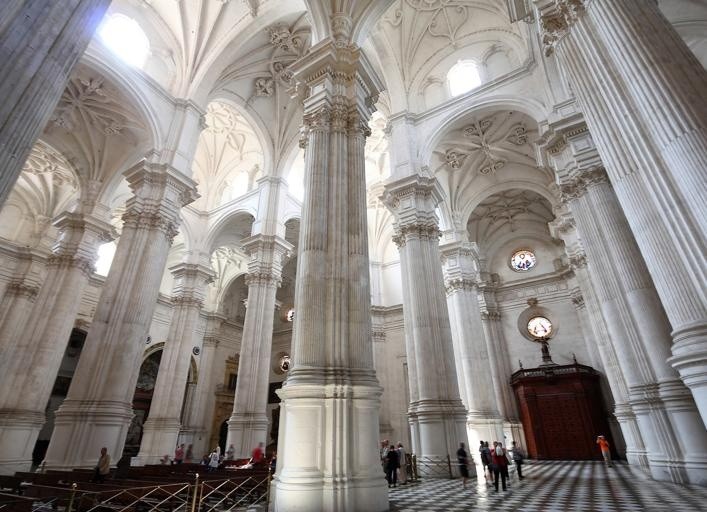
xmin=0 ymin=456 xmax=267 ymax=511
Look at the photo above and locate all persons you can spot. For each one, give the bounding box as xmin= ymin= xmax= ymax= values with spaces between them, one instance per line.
xmin=159 ymin=442 xmax=276 ymax=473
xmin=92 ymin=446 xmax=112 ymax=482
xmin=595 ymin=434 xmax=615 ymax=467
xmin=117 ymin=447 xmax=132 ymax=477
xmin=378 ymin=439 xmax=524 ymax=491
xmin=535 ymin=336 xmax=553 ymax=362
